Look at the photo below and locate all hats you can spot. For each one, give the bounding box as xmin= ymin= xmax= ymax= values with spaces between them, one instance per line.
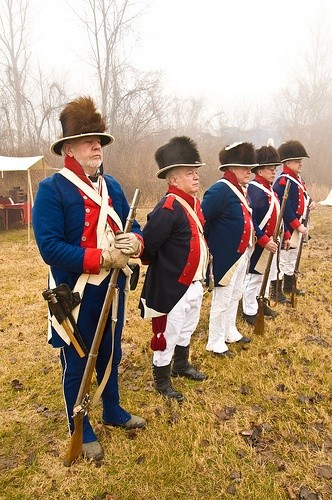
xmin=279 ymin=140 xmax=310 ymax=162
xmin=217 ymin=140 xmax=259 ymax=172
xmin=154 ymin=135 xmax=206 ymax=179
xmin=252 ymin=145 xmax=281 ymax=169
xmin=51 ymin=97 xmax=115 ymax=157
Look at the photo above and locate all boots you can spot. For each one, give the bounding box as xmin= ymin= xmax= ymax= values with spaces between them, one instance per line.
xmin=172 ymin=344 xmax=205 ymax=381
xmin=152 ymin=361 xmax=184 ymax=401
xmin=270 ymin=279 xmax=291 ymax=304
xmin=283 ymin=273 xmax=300 ymax=293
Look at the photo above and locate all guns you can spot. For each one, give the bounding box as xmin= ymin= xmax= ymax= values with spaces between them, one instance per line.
xmin=291 ymin=198 xmax=312 ymax=306
xmin=63 ymin=187 xmax=141 ymax=467
xmin=254 ymin=179 xmax=292 ymax=334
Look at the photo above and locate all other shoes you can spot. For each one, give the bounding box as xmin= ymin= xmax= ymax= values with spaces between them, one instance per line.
xmin=224 ymin=334 xmax=250 ymax=345
xmin=205 ymin=346 xmax=234 ymax=360
xmin=242 ymin=311 xmax=258 ymax=325
xmin=68 ymin=427 xmax=104 ymax=460
xmin=102 ymin=408 xmax=147 ymax=430
xmin=264 ymin=304 xmax=277 ymax=316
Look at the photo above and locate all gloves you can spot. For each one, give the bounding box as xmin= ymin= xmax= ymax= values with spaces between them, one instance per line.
xmin=114 ymin=230 xmax=140 ymax=255
xmin=100 ymin=243 xmax=130 ymax=268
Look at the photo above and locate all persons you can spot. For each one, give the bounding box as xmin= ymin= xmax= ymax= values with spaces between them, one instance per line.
xmin=31 ymin=94 xmax=146 ymax=460
xmin=201 ymin=142 xmax=256 ymax=359
xmin=138 ymin=135 xmax=209 ymax=400
xmin=272 ymin=139 xmax=316 ymax=305
xmin=246 ymin=145 xmax=291 ymax=324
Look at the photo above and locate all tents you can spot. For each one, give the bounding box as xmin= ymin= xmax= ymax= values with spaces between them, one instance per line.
xmin=0 ymin=155 xmax=62 ymax=245
